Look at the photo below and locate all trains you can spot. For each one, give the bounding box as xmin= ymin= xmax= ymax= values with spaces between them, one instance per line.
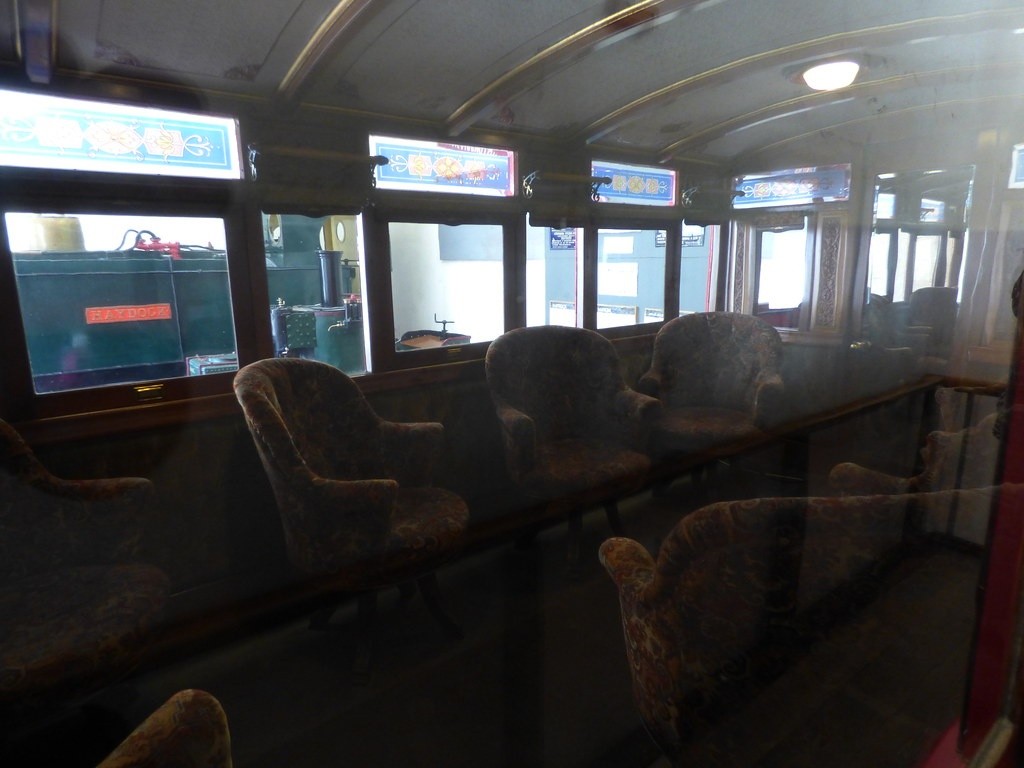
xmin=11 ymin=211 xmax=366 ymax=393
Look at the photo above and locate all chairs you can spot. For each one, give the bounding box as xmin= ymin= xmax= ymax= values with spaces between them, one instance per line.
xmin=0 ymin=418 xmax=181 ymax=713
xmin=233 ymin=358 xmax=474 ymax=636
xmin=640 ymin=310 xmax=787 ymax=507
xmin=485 ymin=327 xmax=665 ymax=567
xmin=94 ymin=689 xmax=233 ymax=768
xmin=921 ymin=383 xmax=1009 ymax=469
xmin=829 ymin=411 xmax=1006 ymax=548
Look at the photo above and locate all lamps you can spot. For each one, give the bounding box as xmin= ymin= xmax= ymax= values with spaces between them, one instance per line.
xmin=802 ymin=58 xmax=861 ymax=94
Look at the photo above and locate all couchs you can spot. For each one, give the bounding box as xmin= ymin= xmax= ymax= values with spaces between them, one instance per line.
xmin=908 ymin=285 xmax=958 ymax=357
xmin=868 ymin=308 xmax=931 ymax=399
xmin=869 ymin=293 xmax=933 ymax=359
xmin=598 ymin=497 xmax=1024 ymax=768
